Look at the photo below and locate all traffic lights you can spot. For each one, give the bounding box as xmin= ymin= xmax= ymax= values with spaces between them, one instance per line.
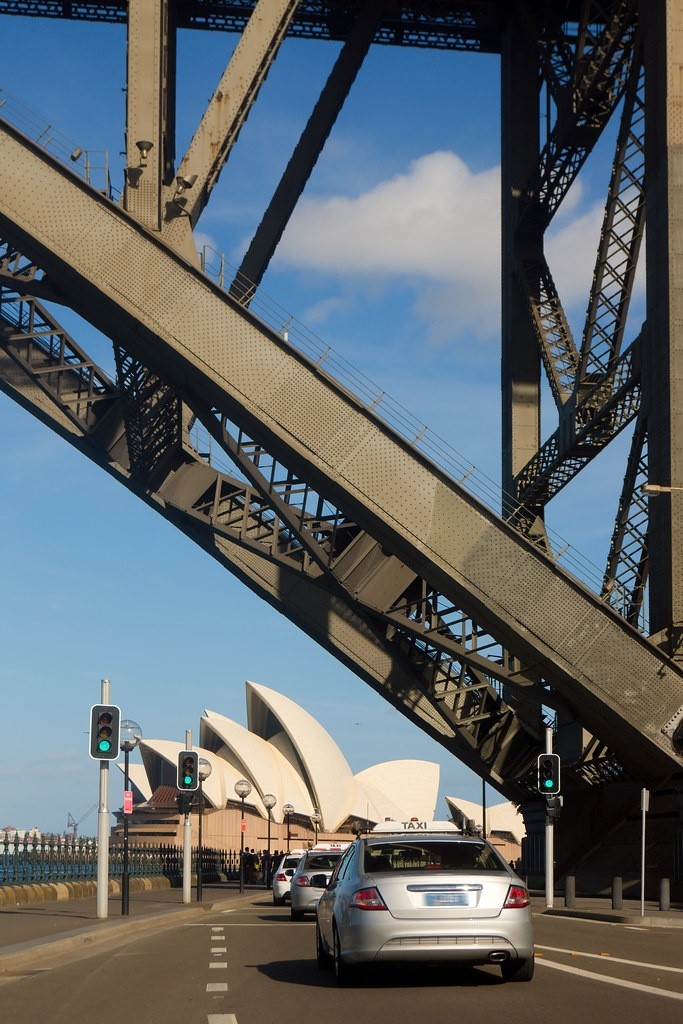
xmin=537 ymin=753 xmax=560 ymax=796
xmin=546 ymin=797 xmax=562 ymax=819
xmin=177 ymin=750 xmax=200 ymax=791
xmin=89 ymin=704 xmax=120 ymax=761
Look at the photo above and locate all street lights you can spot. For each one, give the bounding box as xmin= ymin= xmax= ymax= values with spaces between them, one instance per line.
xmin=311 ymin=813 xmax=322 ymax=845
xmin=234 ymin=780 xmax=253 ymax=893
xmin=197 ymin=758 xmax=212 ymax=902
xmin=120 ymin=720 xmax=143 ymax=915
xmin=262 ymin=794 xmax=277 ymax=889
xmin=282 ymin=803 xmax=295 ymax=852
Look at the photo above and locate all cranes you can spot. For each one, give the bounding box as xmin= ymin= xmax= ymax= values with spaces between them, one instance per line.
xmin=67 ymin=801 xmax=99 ymax=841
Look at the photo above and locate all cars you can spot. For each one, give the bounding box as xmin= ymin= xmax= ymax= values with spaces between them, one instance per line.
xmin=310 ymin=819 xmax=536 ymax=984
xmin=285 ymin=842 xmax=350 ymax=921
xmin=271 ymin=848 xmax=309 ymax=906
